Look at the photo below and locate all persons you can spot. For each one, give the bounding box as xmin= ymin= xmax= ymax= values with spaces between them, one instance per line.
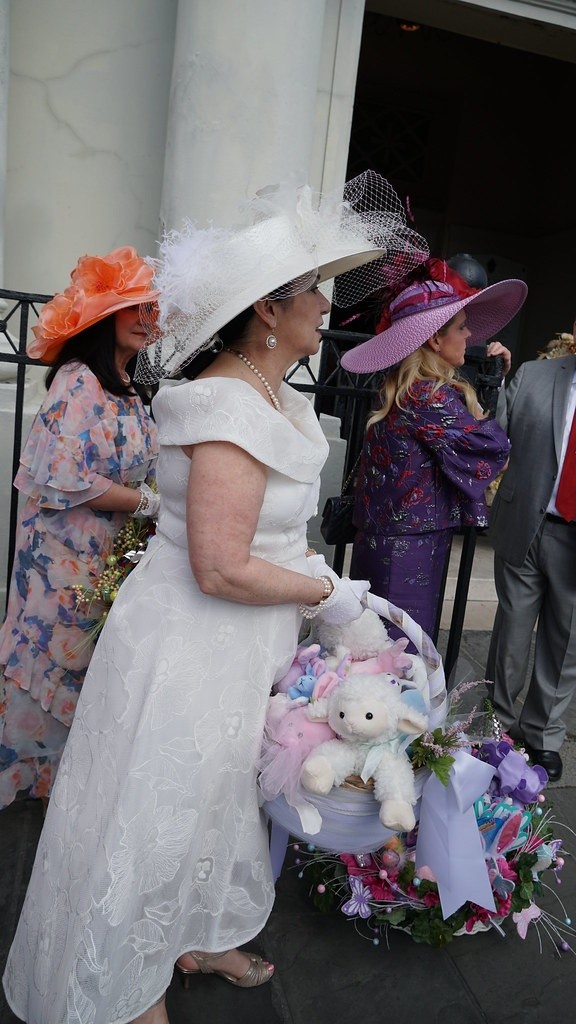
xmin=0 ymin=250 xmax=162 ymax=812
xmin=0 ymin=273 xmax=387 ymax=1024
xmin=348 ymin=309 xmax=512 ymax=667
xmin=478 ymin=338 xmax=576 ymax=781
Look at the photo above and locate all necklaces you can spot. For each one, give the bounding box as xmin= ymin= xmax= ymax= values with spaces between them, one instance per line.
xmin=121 ymin=371 xmax=131 ymax=383
xmin=226 ymin=348 xmax=281 ymax=413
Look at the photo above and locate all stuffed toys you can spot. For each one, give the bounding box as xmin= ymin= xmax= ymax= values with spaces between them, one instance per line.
xmin=255 ymin=606 xmax=431 ymax=804
xmin=301 ymin=673 xmax=428 ymax=833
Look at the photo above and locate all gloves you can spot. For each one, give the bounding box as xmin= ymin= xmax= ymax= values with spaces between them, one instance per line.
xmin=127 ymin=481 xmax=161 ymax=519
xmin=297 ymin=554 xmax=371 ymax=628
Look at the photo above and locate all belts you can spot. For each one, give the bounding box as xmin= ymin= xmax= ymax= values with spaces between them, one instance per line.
xmin=543 ymin=513 xmax=576 ymax=525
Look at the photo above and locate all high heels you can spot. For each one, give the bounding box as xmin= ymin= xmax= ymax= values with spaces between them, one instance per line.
xmin=175 ymin=950 xmax=275 ymax=989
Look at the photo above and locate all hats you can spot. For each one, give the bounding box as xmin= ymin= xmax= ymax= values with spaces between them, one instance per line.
xmin=339 ymin=195 xmax=528 ymax=374
xmin=25 ymin=246 xmax=162 ymax=364
xmin=536 ymin=333 xmax=576 ymax=360
xmin=131 ymin=185 xmax=387 ymax=386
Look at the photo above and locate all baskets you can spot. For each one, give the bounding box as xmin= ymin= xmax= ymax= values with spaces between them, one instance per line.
xmin=257 ymin=591 xmax=472 ymax=855
xmin=382 ymin=872 xmax=538 ymax=936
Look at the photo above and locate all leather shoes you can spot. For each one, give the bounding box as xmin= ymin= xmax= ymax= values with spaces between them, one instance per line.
xmin=532 ymin=750 xmax=562 ymax=781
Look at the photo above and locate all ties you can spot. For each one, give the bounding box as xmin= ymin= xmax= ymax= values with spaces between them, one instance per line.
xmin=554 ymin=407 xmax=576 ymax=522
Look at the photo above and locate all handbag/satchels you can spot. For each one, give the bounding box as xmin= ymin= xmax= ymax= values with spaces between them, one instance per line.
xmin=320 ymin=496 xmax=356 ymax=545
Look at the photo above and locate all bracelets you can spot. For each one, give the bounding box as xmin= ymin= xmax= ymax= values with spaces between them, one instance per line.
xmin=298 ymin=578 xmax=330 ymax=620
xmin=304 ymin=548 xmax=317 ymax=555
xmin=134 ymin=490 xmax=150 ymax=515
